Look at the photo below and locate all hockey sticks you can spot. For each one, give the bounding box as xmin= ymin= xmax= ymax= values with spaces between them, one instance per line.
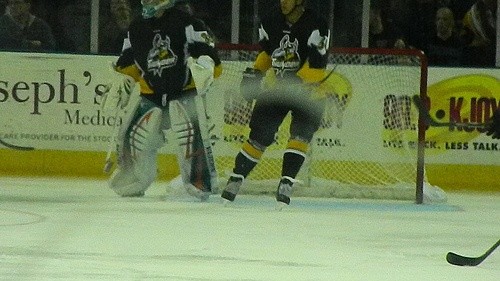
xmin=103 ymin=83 xmax=124 ymax=176
xmin=445 ymin=240 xmax=500 ymax=266
xmin=411 ymin=93 xmax=500 ymax=131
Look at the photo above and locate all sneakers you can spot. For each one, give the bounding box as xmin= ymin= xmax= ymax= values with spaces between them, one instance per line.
xmin=221 ymin=179 xmax=242 ymax=206
xmin=276 ymin=183 xmax=292 ymax=211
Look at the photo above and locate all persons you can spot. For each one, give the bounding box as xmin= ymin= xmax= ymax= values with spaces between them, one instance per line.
xmin=108 ymin=0 xmax=222 ymax=202
xmin=0 ymin=0 xmax=497 ymax=69
xmin=218 ymin=0 xmax=331 ymax=211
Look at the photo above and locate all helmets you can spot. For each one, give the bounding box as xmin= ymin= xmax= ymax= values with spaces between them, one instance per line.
xmin=141 ymin=0 xmax=175 ymax=19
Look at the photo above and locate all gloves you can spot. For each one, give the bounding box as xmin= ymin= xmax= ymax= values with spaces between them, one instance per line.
xmin=240 ymin=66 xmax=264 ymax=103
